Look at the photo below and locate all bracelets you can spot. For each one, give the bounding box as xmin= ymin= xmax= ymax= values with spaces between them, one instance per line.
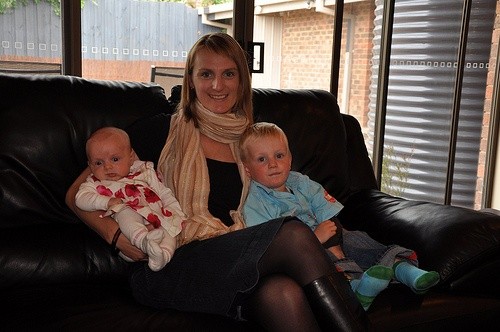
xmin=111 ymin=227 xmax=122 ymax=250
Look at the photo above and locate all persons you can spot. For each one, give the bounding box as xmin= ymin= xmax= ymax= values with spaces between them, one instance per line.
xmin=239 ymin=122 xmax=441 ymax=312
xmin=74 ymin=127 xmax=188 ymax=272
xmin=65 ymin=32 xmax=375 ymax=332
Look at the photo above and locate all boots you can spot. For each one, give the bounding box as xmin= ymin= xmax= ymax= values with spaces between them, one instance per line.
xmin=303 ymin=272 xmax=373 ymax=332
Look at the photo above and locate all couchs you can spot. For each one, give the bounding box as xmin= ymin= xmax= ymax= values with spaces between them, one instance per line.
xmin=1 ymin=75 xmax=500 ymax=332
